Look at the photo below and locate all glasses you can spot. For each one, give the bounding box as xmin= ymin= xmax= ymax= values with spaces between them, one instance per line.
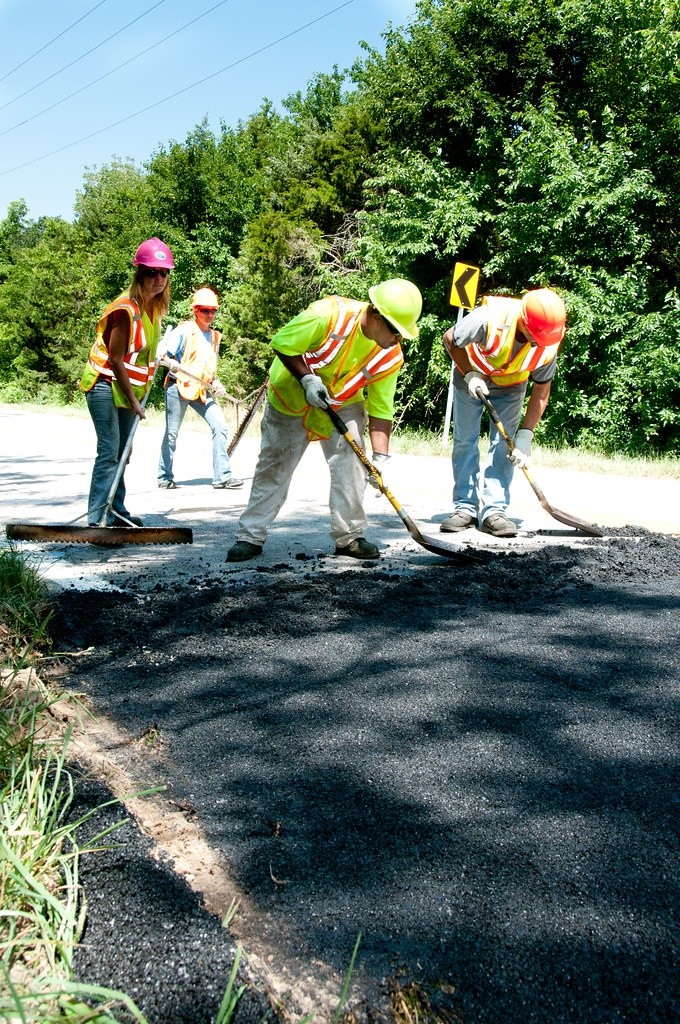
xmin=196 ymin=307 xmax=218 ymax=314
xmin=142 ymin=268 xmax=167 ymax=278
xmin=370 ymin=308 xmax=400 ymax=337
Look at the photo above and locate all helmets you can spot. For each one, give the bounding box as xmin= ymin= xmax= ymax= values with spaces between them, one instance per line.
xmin=190 ymin=288 xmax=218 ymax=307
xmin=134 ymin=237 xmax=175 ymax=270
xmin=368 ymin=278 xmax=423 ymax=339
xmin=523 ymin=289 xmax=566 ymax=347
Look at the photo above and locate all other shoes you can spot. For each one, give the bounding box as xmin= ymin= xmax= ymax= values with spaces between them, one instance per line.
xmin=213 ymin=478 xmax=244 ymax=488
xmin=228 ymin=540 xmax=263 ymax=561
xmin=479 ymin=513 xmax=517 ymax=536
xmin=111 ymin=517 xmax=144 ymax=527
xmin=158 ymin=480 xmax=176 ymax=489
xmin=335 ymin=538 xmax=380 ymax=559
xmin=440 ymin=510 xmax=478 ymax=532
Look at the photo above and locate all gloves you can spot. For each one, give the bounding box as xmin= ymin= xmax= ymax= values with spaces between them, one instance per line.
xmin=463 ymin=371 xmax=489 ymax=402
xmin=299 ymin=373 xmax=334 ymax=410
xmin=505 ymin=428 xmax=536 ymax=469
xmin=364 ymin=452 xmax=391 ymax=498
xmin=212 ymin=379 xmax=226 ymax=398
xmin=163 ymin=357 xmax=180 ymax=373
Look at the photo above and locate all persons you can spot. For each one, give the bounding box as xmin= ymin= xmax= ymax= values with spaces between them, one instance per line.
xmin=444 ymin=288 xmax=572 ymax=536
xmin=77 ymin=237 xmax=175 ymax=525
xmin=159 ymin=288 xmax=244 ymax=490
xmin=229 ymin=277 xmax=423 ymax=561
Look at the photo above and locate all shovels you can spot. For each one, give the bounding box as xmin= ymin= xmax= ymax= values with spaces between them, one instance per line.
xmin=317 ymin=397 xmax=490 ymax=567
xmin=475 ymin=387 xmax=604 ymax=536
